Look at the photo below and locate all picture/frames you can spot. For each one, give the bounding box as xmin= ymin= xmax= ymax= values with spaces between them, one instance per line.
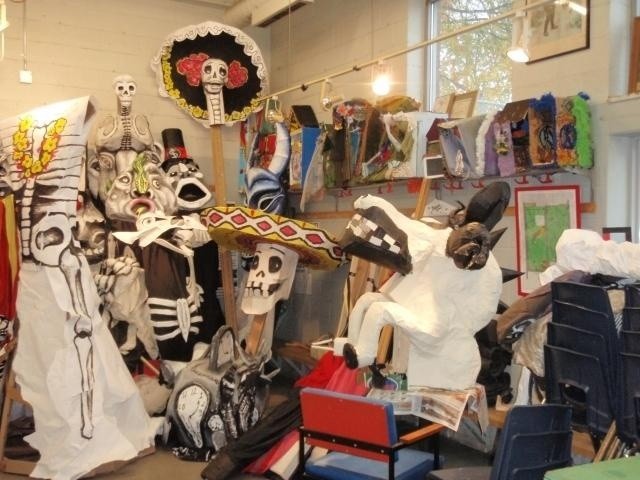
xmin=524 ymin=1 xmax=591 ymax=67
xmin=514 ymin=184 xmax=581 ymax=299
xmin=431 ymin=91 xmax=478 ymax=120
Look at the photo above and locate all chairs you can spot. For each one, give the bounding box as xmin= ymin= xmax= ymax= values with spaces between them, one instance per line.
xmin=289 ymin=384 xmax=448 ymax=479
xmin=490 ymin=282 xmax=639 ymax=480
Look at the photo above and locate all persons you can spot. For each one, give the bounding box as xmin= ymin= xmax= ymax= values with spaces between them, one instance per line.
xmin=543 ymin=4 xmax=561 ymax=34
